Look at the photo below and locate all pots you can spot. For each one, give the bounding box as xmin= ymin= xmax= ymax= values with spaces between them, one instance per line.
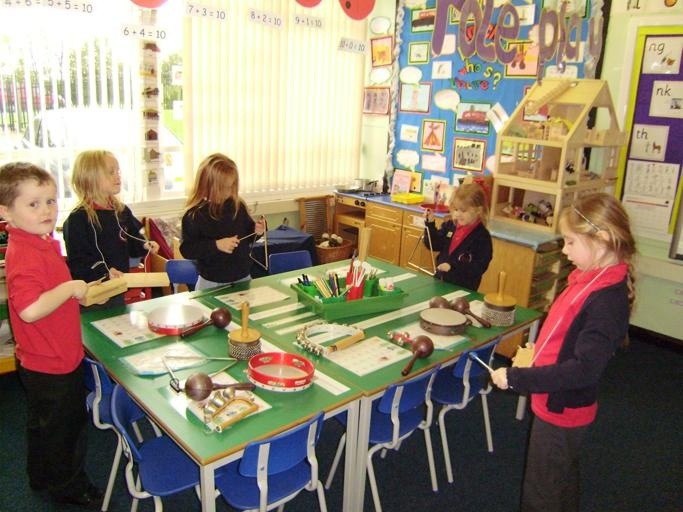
xmin=353 ymin=177 xmax=379 ymax=192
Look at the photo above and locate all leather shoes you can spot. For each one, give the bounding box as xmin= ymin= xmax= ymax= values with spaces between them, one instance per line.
xmin=28 ymin=475 xmax=111 ymax=512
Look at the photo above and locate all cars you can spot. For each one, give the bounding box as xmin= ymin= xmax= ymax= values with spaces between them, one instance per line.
xmin=22 ymin=105 xmax=189 ymax=199
xmin=0 ymin=125 xmax=69 ymax=206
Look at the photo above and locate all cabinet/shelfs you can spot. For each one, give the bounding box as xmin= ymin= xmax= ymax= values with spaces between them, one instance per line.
xmin=332 ymin=192 xmax=564 ymax=360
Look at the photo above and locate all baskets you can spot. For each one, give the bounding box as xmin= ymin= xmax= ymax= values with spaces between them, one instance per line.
xmin=313 ymin=238 xmax=353 ymax=264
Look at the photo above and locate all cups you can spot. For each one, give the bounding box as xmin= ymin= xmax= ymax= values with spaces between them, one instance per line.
xmin=298 ymin=274 xmax=402 ymax=304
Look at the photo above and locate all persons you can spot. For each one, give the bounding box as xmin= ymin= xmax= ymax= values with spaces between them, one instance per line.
xmin=179 ymin=152 xmax=268 ymax=291
xmin=1 ymin=161 xmax=106 ymax=506
xmin=422 ymin=175 xmax=493 ymax=293
xmin=64 ymin=150 xmax=160 ymax=314
xmin=488 ymin=191 xmax=637 ymax=512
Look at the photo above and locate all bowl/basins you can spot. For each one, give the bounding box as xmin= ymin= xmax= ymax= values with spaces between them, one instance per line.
xmin=334 ymin=183 xmax=360 ymax=194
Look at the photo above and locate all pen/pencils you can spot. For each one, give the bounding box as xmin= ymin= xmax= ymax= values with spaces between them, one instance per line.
xmin=297 ymin=249 xmax=378 ymax=299
